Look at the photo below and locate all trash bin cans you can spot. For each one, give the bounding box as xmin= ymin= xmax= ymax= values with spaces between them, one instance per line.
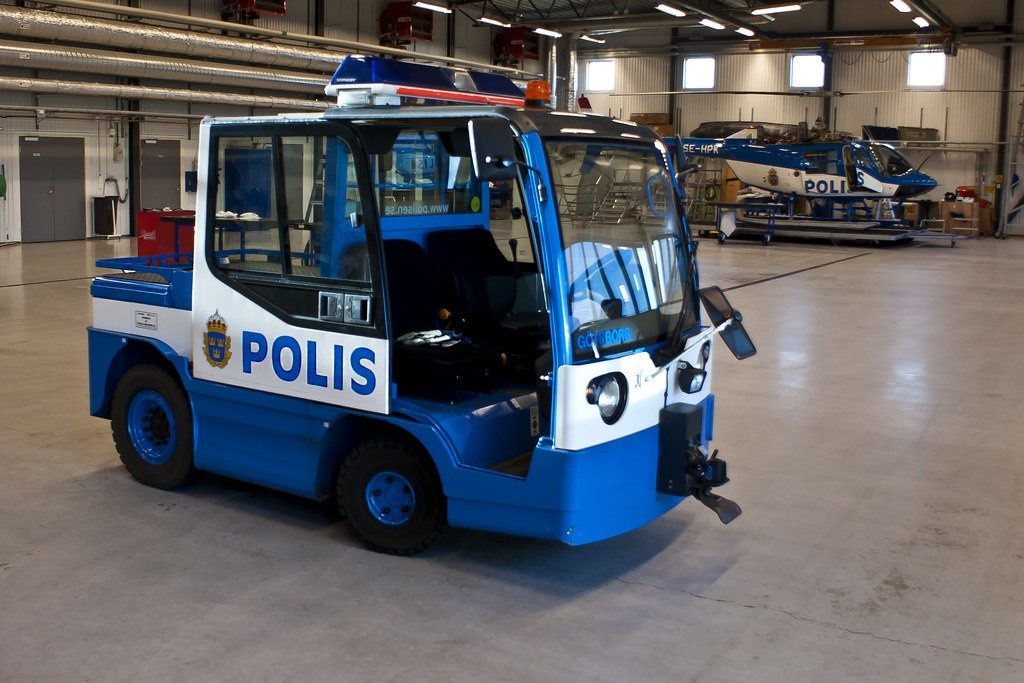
xmin=93 ymin=195 xmax=120 ymax=236
xmin=136 ymin=209 xmax=196 ymax=266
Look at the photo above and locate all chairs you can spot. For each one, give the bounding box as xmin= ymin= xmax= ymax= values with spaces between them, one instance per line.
xmin=341 ymin=239 xmax=497 ymax=383
xmin=421 ymin=227 xmax=549 ymax=351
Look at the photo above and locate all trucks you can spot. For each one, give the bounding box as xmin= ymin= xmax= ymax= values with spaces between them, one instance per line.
xmin=82 ymin=53 xmax=754 ymax=561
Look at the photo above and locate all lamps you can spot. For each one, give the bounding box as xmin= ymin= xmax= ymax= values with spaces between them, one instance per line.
xmin=411 ymin=0 xmax=452 ymax=14
xmin=531 ymin=26 xmax=563 ymax=38
xmin=750 ymin=1 xmax=802 ymax=16
xmin=888 ymin=0 xmax=933 ymax=28
xmin=653 ymin=1 xmax=687 ymax=18
xmin=733 ymin=26 xmax=757 ymax=38
xmin=476 ymin=15 xmax=512 ymax=28
xmin=697 ymin=16 xmax=726 ymax=30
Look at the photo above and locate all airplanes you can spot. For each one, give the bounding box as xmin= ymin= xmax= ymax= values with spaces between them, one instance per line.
xmin=653 ymin=124 xmax=941 ymax=229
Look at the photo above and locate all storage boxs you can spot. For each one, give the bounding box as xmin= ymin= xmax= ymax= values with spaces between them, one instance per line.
xmin=629 ymin=112 xmax=670 ymax=125
xmin=861 ymin=125 xmax=897 ymax=140
xmin=896 ymin=125 xmax=939 ymax=144
xmin=647 ymin=124 xmax=674 ymax=137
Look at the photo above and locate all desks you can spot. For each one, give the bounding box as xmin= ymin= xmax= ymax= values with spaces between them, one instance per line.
xmin=160 ymin=214 xmax=304 ymax=264
